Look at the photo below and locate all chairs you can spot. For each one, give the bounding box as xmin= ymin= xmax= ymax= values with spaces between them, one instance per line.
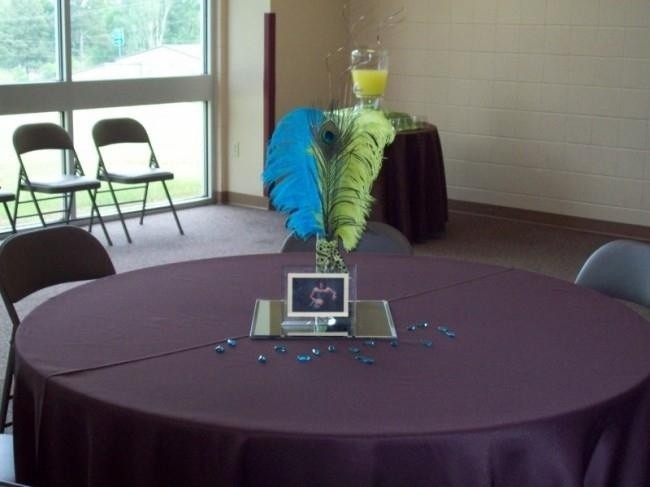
xmin=575 ymin=239 xmax=650 ymax=304
xmin=93 ymin=118 xmax=185 ymax=244
xmin=0 ymin=227 xmax=116 ymax=434
xmin=1 ymin=193 xmax=20 ymax=232
xmin=11 ymin=123 xmax=115 ymax=249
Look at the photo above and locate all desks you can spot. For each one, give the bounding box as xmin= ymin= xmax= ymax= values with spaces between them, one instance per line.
xmin=13 ymin=252 xmax=650 ymax=487
xmin=369 ymin=120 xmax=449 ymax=242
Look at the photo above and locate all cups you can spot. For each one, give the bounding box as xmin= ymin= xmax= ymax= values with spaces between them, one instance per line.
xmin=388 ymin=114 xmax=429 ymax=131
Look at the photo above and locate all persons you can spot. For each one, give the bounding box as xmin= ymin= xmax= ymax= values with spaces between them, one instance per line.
xmin=305 ymin=280 xmax=338 ymax=311
